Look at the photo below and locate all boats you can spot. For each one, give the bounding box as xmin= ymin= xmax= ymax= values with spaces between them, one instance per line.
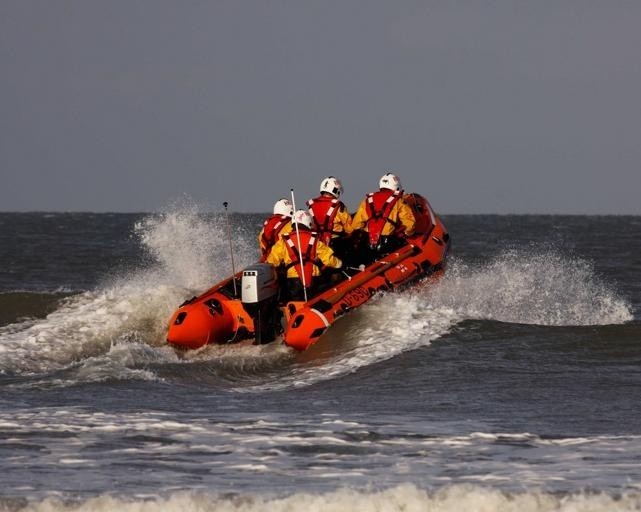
xmin=166 ymin=193 xmax=448 ymax=350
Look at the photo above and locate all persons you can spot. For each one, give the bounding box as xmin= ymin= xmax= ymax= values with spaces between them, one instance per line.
xmin=304 ymin=178 xmax=354 ymax=248
xmin=352 ymin=171 xmax=416 ymax=258
xmin=257 ymin=198 xmax=297 ymax=260
xmin=265 ymin=208 xmax=344 ymax=303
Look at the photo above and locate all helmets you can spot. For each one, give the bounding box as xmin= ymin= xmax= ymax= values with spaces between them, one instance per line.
xmin=319 ymin=177 xmax=343 ymax=199
xmin=292 ymin=209 xmax=315 ymax=230
xmin=379 ymin=173 xmax=403 ymax=192
xmin=273 ymin=197 xmax=295 ymax=217
